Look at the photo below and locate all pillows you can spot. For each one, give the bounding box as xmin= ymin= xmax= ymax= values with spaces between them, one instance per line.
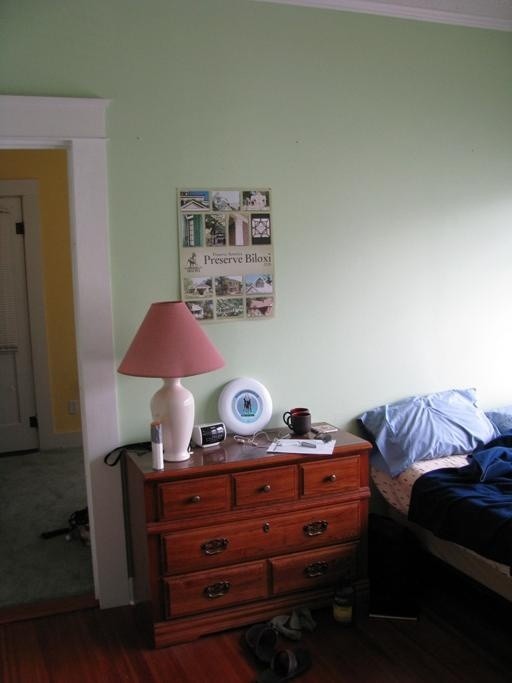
xmin=357 ymin=388 xmax=512 ymax=480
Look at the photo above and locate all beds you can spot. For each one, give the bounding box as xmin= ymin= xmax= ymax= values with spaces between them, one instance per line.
xmin=371 ymin=436 xmax=512 ymax=604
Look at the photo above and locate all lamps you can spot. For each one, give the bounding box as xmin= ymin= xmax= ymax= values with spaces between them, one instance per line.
xmin=116 ymin=301 xmax=225 ymax=462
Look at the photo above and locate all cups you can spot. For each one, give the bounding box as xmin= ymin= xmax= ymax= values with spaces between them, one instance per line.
xmin=287 ymin=412 xmax=311 ymax=434
xmin=283 ymin=408 xmax=309 ymax=426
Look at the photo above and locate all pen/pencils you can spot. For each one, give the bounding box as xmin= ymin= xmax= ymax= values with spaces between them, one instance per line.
xmin=302 ymin=442 xmax=316 ymax=448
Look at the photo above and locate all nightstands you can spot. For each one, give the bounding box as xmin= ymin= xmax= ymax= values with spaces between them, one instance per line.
xmin=124 ymin=422 xmax=373 ymax=650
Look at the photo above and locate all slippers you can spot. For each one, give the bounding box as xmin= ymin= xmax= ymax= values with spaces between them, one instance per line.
xmin=246 ymin=621 xmax=281 ymax=664
xmin=256 ymin=648 xmax=312 ymax=682
